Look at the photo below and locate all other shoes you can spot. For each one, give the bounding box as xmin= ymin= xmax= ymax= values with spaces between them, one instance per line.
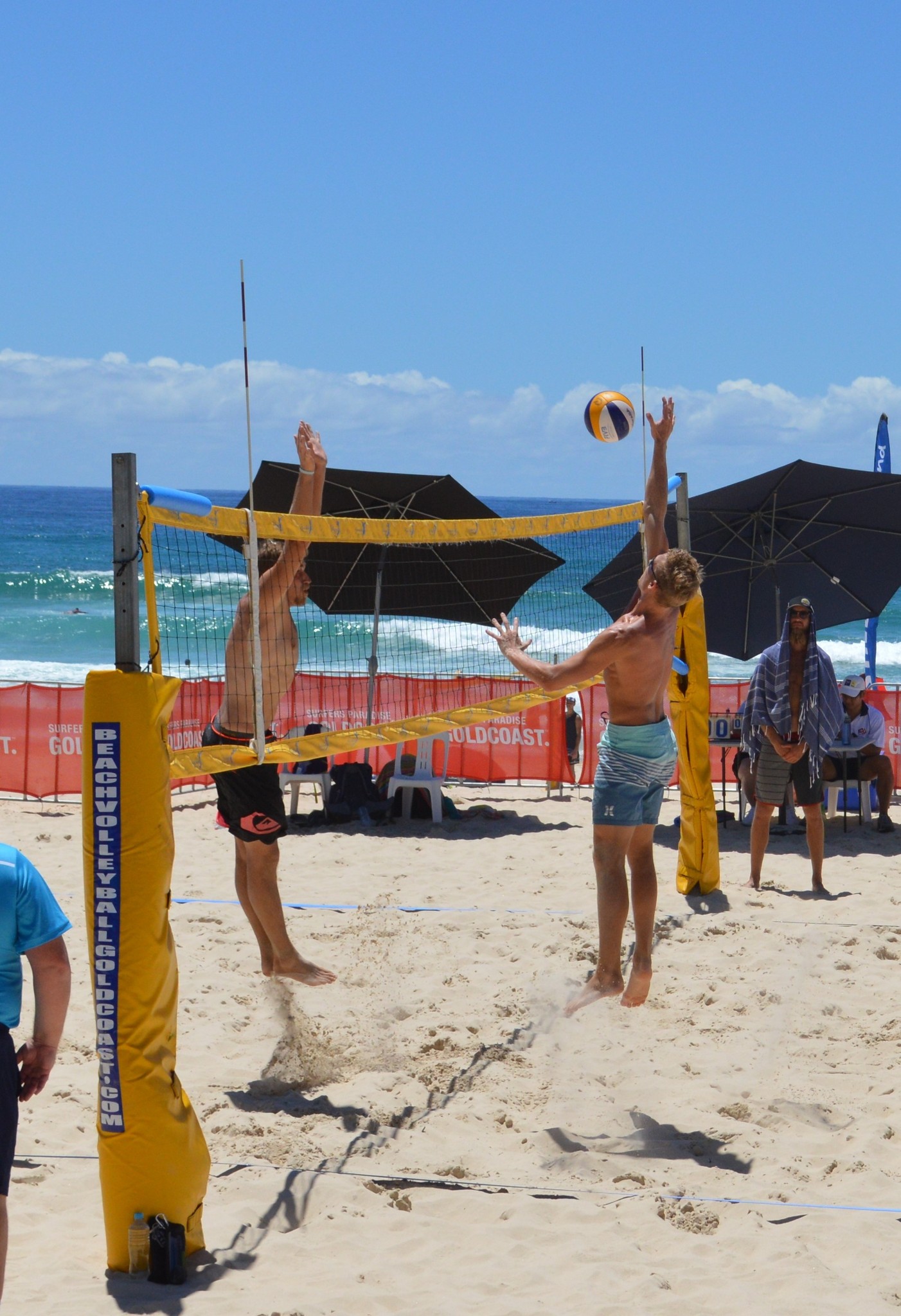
xmin=741 ymin=806 xmax=755 ymax=825
xmin=877 ymin=812 xmax=895 ymax=831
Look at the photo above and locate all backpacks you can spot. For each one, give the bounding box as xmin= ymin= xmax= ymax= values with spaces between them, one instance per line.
xmin=375 ymin=752 xmax=447 ymax=821
xmin=326 ymin=760 xmax=386 ymax=820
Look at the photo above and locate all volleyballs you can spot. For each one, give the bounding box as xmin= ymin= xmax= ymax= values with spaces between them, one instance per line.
xmin=584 ymin=390 xmax=635 ymax=442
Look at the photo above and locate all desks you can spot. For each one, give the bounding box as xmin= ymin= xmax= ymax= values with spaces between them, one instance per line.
xmin=708 ymin=738 xmax=876 ymax=834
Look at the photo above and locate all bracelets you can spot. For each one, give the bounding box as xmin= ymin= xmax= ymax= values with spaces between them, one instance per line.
xmin=299 ymin=467 xmax=315 ymax=475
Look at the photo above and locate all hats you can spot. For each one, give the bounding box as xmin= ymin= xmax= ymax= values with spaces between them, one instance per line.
xmin=786 ymin=596 xmax=814 ymax=614
xmin=839 ymin=674 xmax=866 ymax=698
xmin=566 ymin=697 xmax=576 ymax=703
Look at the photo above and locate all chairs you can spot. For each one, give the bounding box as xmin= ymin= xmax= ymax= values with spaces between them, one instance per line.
xmin=279 ymin=726 xmax=334 ymax=818
xmin=739 ymin=781 xmax=794 ymax=821
xmin=386 ymin=732 xmax=450 ymax=824
xmin=822 ymin=780 xmax=873 ymax=823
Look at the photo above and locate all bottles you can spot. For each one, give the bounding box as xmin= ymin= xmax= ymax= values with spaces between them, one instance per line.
xmin=128 ymin=1212 xmax=150 ymax=1280
xmin=444 ymin=796 xmax=461 ymax=819
xmin=674 ymin=816 xmax=681 ymax=827
xmin=358 ymin=806 xmax=371 ymax=826
xmin=841 ymin=714 xmax=852 ymax=746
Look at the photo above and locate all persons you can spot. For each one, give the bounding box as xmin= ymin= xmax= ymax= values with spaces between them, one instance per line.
xmin=485 ymin=396 xmax=706 ymax=1017
xmin=201 ymin=422 xmax=338 ymax=986
xmin=0 ymin=842 xmax=74 ymax=1300
xmin=739 ymin=596 xmax=848 ymax=894
xmin=733 ymin=675 xmax=896 ymax=832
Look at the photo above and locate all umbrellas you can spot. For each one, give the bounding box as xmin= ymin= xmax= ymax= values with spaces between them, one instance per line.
xmin=206 ymin=460 xmax=563 ymax=763
xmin=581 ymin=458 xmax=901 ymax=663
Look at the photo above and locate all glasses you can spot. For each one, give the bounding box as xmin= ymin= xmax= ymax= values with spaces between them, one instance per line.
xmin=647 ymin=557 xmax=661 ymax=590
xmin=790 ymin=609 xmax=811 ymax=619
